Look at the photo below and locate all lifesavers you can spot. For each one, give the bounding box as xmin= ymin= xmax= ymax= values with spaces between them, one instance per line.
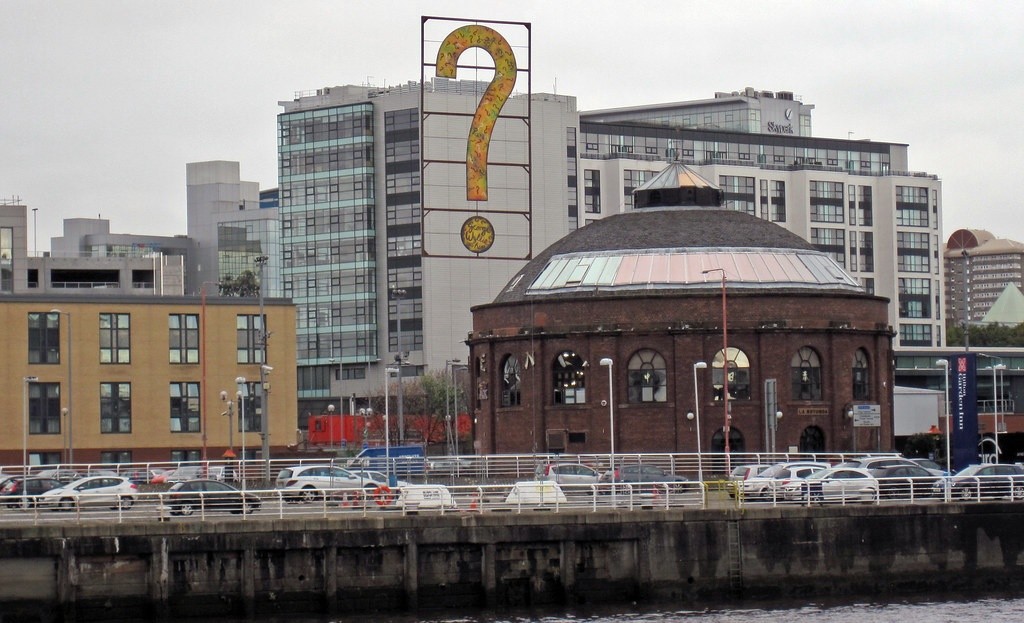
xmin=374 ymin=486 xmax=392 ymax=506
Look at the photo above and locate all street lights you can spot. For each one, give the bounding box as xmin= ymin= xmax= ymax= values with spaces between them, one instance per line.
xmin=694 ymin=362 xmax=707 ymax=482
xmin=600 ymin=358 xmax=616 ymax=510
xmin=384 ymin=368 xmax=400 ymax=486
xmin=936 ymin=359 xmax=951 ymax=502
xmin=993 ymin=364 xmax=1007 ymax=464
xmin=235 ymin=391 xmax=247 ymax=520
xmin=454 ymin=367 xmax=467 ymax=456
xmin=23 ymin=376 xmax=38 ymax=512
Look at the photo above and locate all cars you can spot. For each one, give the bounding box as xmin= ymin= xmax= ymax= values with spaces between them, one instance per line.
xmin=783 ymin=467 xmax=879 ymax=504
xmin=598 ymin=464 xmax=690 ymax=494
xmin=842 ymin=457 xmax=952 ymax=478
xmin=0 ymin=466 xmax=261 ymax=515
xmin=534 ymin=463 xmax=603 ymax=496
xmin=736 ymin=461 xmax=833 ymax=500
xmin=932 ymin=464 xmax=1024 ymax=501
xmin=726 ymin=464 xmax=771 ymax=498
xmin=271 ymin=465 xmax=413 ymax=502
xmin=870 ymin=465 xmax=943 ymax=500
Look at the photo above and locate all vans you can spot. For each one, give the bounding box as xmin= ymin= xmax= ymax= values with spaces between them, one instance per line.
xmin=348 ymin=445 xmax=427 ymax=479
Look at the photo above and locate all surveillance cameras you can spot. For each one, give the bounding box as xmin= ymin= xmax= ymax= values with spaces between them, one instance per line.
xmin=262 ymin=365 xmax=274 ymax=372
xmin=582 ymin=361 xmax=588 ymax=367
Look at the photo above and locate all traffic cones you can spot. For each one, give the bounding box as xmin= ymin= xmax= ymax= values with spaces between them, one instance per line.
xmin=342 ymin=492 xmax=350 ymax=507
